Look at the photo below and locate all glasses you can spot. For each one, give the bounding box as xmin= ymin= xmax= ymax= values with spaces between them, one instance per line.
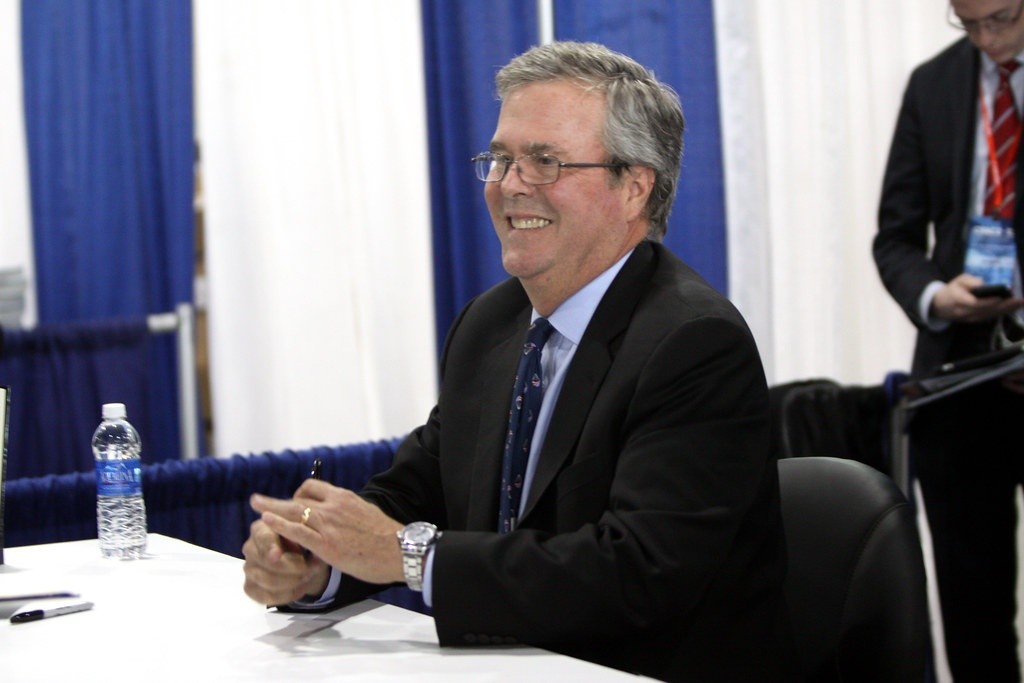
xmin=945 ymin=2 xmax=1024 ymax=32
xmin=471 ymin=149 xmax=630 ymax=185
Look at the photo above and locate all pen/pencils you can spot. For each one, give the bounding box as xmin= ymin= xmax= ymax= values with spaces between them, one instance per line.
xmin=10 ymin=599 xmax=98 ymax=622
xmin=298 ymin=452 xmax=327 ymax=568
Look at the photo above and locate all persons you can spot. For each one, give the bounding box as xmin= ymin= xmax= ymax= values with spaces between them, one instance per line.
xmin=243 ymin=42 xmax=787 ymax=683
xmin=872 ymin=0 xmax=1024 ymax=683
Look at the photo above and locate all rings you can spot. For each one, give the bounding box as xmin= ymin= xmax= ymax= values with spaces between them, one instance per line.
xmin=301 ymin=506 xmax=311 ymax=525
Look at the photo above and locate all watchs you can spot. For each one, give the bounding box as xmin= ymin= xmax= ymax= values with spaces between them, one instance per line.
xmin=396 ymin=521 xmax=443 ymax=592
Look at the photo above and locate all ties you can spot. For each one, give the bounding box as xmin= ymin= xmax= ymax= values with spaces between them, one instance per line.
xmin=982 ymin=59 xmax=1024 ymax=221
xmin=496 ymin=316 xmax=556 ymax=535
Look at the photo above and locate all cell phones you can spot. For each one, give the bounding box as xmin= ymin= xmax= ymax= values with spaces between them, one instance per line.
xmin=969 ymin=283 xmax=1014 ymax=298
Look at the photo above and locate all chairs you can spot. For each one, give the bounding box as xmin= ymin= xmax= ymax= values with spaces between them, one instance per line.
xmin=777 ymin=456 xmax=937 ymax=683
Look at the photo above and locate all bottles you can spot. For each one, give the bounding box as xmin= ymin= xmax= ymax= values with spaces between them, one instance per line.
xmin=91 ymin=403 xmax=148 ymax=559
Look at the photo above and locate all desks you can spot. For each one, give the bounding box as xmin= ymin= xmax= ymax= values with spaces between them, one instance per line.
xmin=0 ymin=534 xmax=668 ymax=683
